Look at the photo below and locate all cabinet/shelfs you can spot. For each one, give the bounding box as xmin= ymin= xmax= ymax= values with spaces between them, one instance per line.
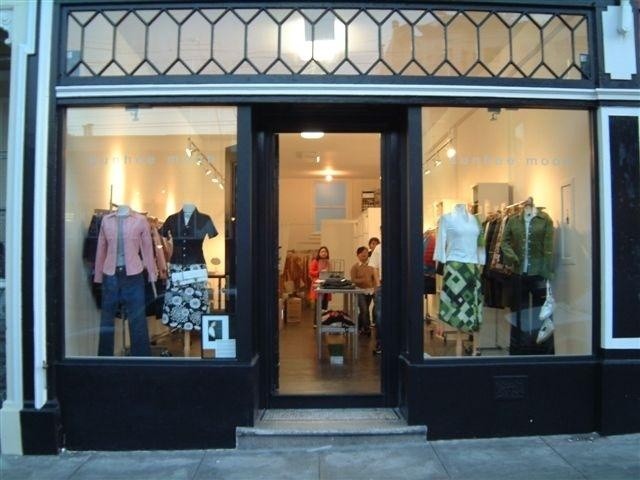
xmin=472 ymin=181 xmax=511 ymax=349
xmin=352 ymin=207 xmax=385 ymax=329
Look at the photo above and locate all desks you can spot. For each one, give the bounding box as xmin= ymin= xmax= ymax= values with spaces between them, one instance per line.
xmin=315 ymin=281 xmax=362 ymax=363
xmin=206 ymin=272 xmax=225 ymax=313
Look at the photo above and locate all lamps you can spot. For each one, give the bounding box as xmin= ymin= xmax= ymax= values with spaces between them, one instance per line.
xmin=186 ymin=139 xmax=224 ymax=188
xmin=424 ymin=156 xmax=442 ymax=175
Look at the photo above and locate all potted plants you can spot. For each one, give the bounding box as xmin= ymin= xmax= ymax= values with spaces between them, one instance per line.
xmin=328 ymin=344 xmax=346 ymax=365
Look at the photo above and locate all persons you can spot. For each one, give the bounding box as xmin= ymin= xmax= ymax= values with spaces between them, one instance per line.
xmin=351 ymin=238 xmax=382 ymax=354
xmin=309 ymin=247 xmax=331 ymax=328
xmin=93 ymin=205 xmax=159 ymax=356
xmin=433 ymin=204 xmax=482 ymax=335
xmin=157 ymin=203 xmax=218 ymax=330
xmin=501 ymin=204 xmax=554 ymax=355
xmin=209 ymin=321 xmax=216 ymax=341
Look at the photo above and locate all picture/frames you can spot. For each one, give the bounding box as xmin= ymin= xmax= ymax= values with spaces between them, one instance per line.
xmin=559 ymin=177 xmax=577 ymax=265
